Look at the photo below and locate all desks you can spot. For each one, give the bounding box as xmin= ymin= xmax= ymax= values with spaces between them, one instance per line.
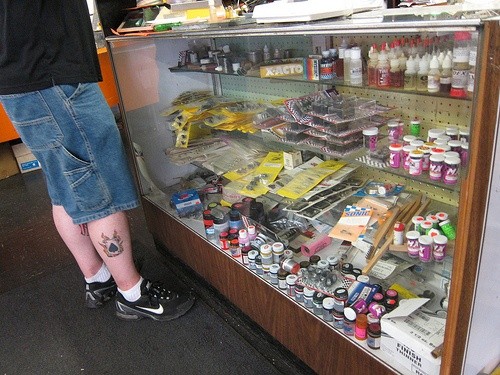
xmin=0 ymin=37 xmax=160 ymax=145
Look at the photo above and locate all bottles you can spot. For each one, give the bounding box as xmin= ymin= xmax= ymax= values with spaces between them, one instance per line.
xmin=320 ymin=39 xmax=362 ymax=83
xmin=362 ymin=119 xmax=469 ymax=184
xmin=278 ymin=269 xmax=289 ymax=290
xmin=287 ymin=255 xmax=399 ymax=349
xmin=226 ymin=9 xmax=232 ymax=18
xmin=367 ymin=32 xmax=479 ymax=98
xmin=203 ymin=196 xmax=294 ymax=285
xmin=208 ymin=45 xmax=240 ymax=71
xmin=248 ymin=44 xmax=291 ymax=65
xmin=280 ymin=258 xmax=300 ymax=275
xmin=394 ymin=212 xmax=456 ymax=262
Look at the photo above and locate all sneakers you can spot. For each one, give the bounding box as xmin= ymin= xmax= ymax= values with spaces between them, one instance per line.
xmin=85 ymin=275 xmax=117 ymax=308
xmin=115 ymin=279 xmax=196 ymax=321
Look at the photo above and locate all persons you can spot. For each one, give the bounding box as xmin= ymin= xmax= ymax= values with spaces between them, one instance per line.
xmin=0 ymin=0 xmax=193 ymax=322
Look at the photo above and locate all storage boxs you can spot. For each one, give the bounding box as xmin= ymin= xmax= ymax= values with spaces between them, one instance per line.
xmin=380 ymin=298 xmax=447 ymax=375
xmin=258 ymin=62 xmax=305 ymax=79
xmin=303 ymin=57 xmax=320 ymax=81
xmin=0 ymin=144 xmax=21 ymax=181
xmin=171 ymin=189 xmax=202 ymax=219
xmin=283 ymin=149 xmax=302 ymax=171
xmin=10 ymin=138 xmax=42 ymax=174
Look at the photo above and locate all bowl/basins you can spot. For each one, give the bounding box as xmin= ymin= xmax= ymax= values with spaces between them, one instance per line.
xmin=242 ymin=13 xmax=253 ymax=18
xmin=236 ymin=9 xmax=246 ymax=16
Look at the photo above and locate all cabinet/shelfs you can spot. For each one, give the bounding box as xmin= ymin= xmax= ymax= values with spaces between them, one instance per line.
xmin=103 ymin=16 xmax=500 ymax=375
